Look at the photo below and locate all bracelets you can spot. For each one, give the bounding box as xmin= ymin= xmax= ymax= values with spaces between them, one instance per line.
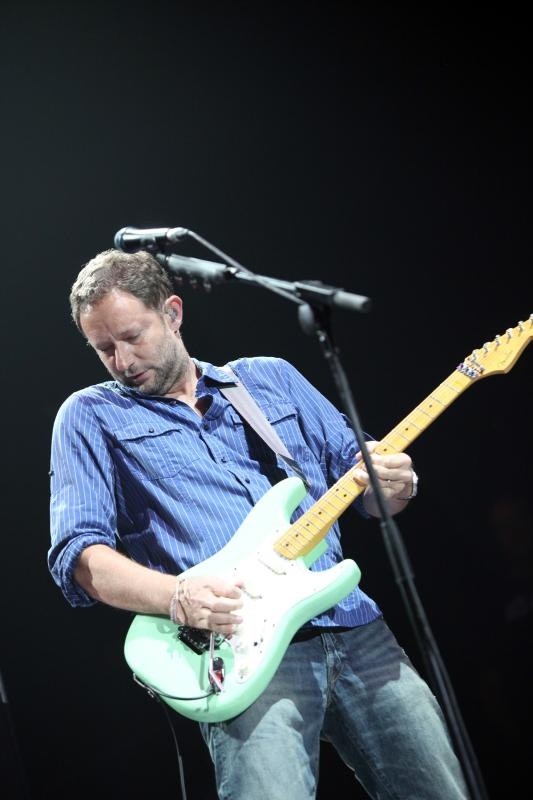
xmin=169 ymin=577 xmax=186 ymax=625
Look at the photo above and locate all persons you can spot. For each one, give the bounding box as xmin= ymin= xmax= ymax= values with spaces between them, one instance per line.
xmin=47 ymin=249 xmax=471 ymax=800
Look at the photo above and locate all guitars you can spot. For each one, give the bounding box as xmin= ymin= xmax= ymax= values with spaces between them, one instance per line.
xmin=123 ymin=312 xmax=533 ymax=723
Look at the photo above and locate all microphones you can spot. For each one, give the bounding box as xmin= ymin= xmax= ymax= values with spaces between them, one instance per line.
xmin=114 ymin=225 xmax=189 ymax=255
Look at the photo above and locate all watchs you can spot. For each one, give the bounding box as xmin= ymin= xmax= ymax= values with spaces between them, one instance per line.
xmin=399 ymin=467 xmax=419 ymax=500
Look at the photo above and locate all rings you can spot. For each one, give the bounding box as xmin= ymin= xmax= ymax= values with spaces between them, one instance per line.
xmin=386 ymin=479 xmax=391 ymax=488
